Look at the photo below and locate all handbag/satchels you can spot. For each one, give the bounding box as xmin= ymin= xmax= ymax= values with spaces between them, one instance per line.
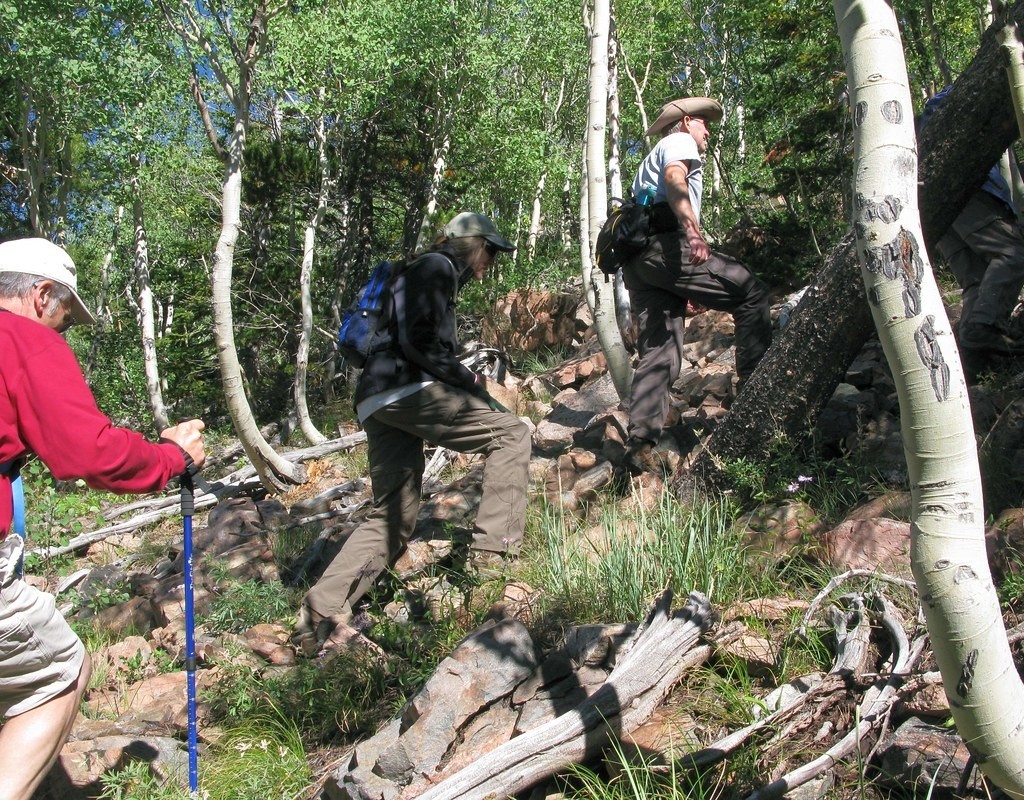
xmin=596 ymin=186 xmax=678 ymax=283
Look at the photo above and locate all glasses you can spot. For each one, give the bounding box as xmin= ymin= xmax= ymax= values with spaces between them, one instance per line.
xmin=691 ymin=119 xmax=708 ymax=128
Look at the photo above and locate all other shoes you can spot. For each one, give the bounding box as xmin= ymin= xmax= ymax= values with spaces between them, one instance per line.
xmin=961 ymin=327 xmax=1024 ymax=356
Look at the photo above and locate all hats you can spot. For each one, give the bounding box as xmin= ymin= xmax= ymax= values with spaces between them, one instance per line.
xmin=0 ymin=238 xmax=97 ymax=325
xmin=645 ymin=97 xmax=723 ymax=136
xmin=444 ymin=211 xmax=518 ymax=252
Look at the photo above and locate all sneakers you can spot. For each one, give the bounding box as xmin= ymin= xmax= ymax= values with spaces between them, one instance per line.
xmin=626 ymin=439 xmax=662 ymax=474
xmin=472 ymin=551 xmax=504 ymax=579
xmin=288 ymin=610 xmax=327 ymax=658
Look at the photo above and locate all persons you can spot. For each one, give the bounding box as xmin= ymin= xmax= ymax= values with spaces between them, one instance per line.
xmin=916 ymin=85 xmax=1023 ymax=354
xmin=623 ymin=96 xmax=773 ymax=479
xmin=299 ymin=212 xmax=532 ymax=658
xmin=0 ymin=237 xmax=207 ymax=800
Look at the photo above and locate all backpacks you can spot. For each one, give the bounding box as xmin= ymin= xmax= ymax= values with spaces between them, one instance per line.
xmin=337 ymin=253 xmax=457 ymax=369
